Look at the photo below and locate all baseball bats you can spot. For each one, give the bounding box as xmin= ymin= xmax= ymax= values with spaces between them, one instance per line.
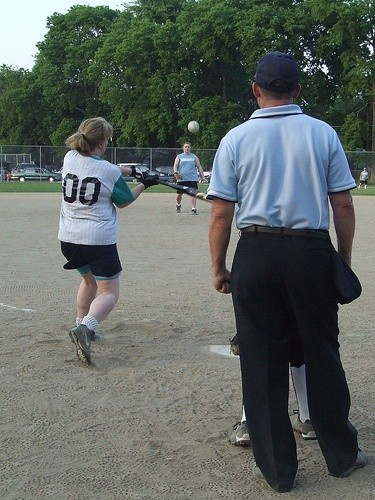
xmin=160 ymin=179 xmax=213 ymax=203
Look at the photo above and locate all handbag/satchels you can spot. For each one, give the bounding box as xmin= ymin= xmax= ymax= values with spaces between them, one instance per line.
xmin=332 ymin=244 xmax=364 ymax=305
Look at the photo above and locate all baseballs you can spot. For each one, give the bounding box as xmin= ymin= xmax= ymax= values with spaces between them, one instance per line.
xmin=187 ymin=120 xmax=200 ymax=133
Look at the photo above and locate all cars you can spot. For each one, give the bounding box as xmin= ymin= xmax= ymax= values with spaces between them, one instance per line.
xmin=6 ymin=167 xmax=62 ymax=183
xmin=197 ymin=171 xmax=212 ymax=184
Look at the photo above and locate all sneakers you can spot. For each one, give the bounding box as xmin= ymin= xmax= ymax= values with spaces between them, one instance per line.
xmin=69 ymin=325 xmax=93 ymax=364
xmin=230 ymin=421 xmax=251 ymax=446
xmin=289 ymin=410 xmax=317 ymax=438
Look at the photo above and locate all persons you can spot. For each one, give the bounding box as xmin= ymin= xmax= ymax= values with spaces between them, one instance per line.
xmin=358 ymin=168 xmax=368 ymax=189
xmin=174 ymin=143 xmax=206 ymax=215
xmin=58 ymin=118 xmax=159 ymax=364
xmin=206 ymin=51 xmax=368 ymax=492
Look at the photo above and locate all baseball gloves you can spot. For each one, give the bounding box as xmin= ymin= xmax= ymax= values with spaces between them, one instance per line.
xmin=228 ymin=333 xmax=240 ymax=356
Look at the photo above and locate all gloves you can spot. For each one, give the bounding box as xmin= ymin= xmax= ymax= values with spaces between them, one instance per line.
xmin=142 ymin=174 xmax=160 ymax=189
xmin=130 ymin=166 xmax=143 ymax=183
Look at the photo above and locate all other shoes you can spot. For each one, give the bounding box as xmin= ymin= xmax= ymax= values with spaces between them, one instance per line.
xmin=191 ymin=209 xmax=197 ymax=214
xmin=254 ymin=464 xmax=279 ymax=493
xmin=176 ymin=204 xmax=181 ymax=212
xmin=328 ymin=448 xmax=367 ymax=477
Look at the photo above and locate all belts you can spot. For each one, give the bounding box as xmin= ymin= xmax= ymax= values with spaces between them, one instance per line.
xmin=241 ymin=225 xmax=329 ymax=239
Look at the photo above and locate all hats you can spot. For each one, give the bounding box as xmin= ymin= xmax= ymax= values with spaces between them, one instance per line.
xmin=255 ymin=50 xmax=300 ymax=91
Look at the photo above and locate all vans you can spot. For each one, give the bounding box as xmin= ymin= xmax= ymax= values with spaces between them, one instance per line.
xmin=117 ymin=162 xmax=160 ymax=178
